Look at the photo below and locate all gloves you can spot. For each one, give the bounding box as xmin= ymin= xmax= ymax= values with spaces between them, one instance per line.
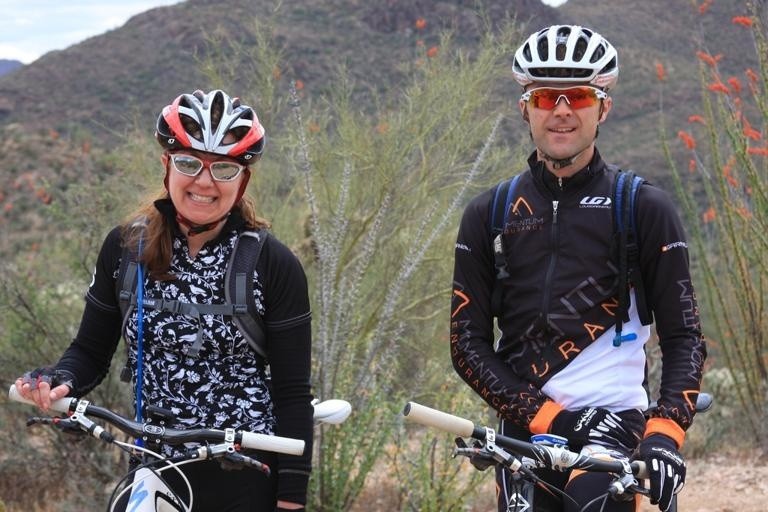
xmin=16 ymin=365 xmax=82 ymax=403
xmin=547 ymin=406 xmax=642 ymax=463
xmin=628 ymin=433 xmax=687 ymax=512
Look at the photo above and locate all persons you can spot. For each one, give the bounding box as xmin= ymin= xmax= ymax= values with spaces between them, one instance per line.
xmin=448 ymin=24 xmax=708 ymax=512
xmin=13 ymin=88 xmax=315 ymax=512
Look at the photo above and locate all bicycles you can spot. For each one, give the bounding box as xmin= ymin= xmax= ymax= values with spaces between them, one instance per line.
xmin=9 ymin=383 xmax=352 ymax=512
xmin=404 ymin=392 xmax=712 ymax=512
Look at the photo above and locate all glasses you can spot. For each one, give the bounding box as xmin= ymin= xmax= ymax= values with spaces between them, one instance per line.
xmin=519 ymin=85 xmax=609 ymax=110
xmin=167 ymin=152 xmax=246 ymax=182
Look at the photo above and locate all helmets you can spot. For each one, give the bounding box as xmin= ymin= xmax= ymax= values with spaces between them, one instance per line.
xmin=511 ymin=22 xmax=620 ymax=87
xmin=156 ymin=88 xmax=266 ymax=166
